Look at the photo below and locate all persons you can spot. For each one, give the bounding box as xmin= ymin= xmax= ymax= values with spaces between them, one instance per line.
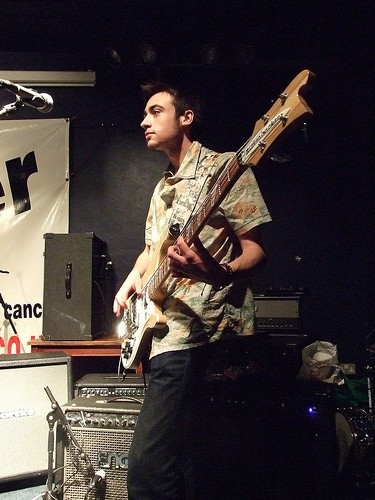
xmin=111 ymin=82 xmax=272 ymax=500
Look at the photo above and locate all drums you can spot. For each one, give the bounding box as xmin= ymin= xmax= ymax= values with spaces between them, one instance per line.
xmin=335 ymin=407 xmax=375 ymax=473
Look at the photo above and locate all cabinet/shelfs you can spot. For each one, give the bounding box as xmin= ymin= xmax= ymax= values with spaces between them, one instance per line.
xmin=0 ymin=351 xmax=72 ymax=484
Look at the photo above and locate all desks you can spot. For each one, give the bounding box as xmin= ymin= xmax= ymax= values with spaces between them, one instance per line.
xmin=27 ymin=338 xmax=143 ymax=375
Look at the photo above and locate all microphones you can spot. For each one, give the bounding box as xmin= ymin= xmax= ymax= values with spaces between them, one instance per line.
xmin=0 ymin=79 xmax=53 ymax=113
xmin=81 ymin=453 xmax=105 ymax=490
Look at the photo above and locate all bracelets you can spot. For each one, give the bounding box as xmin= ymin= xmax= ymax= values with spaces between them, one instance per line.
xmin=221 ymin=262 xmax=236 ymax=286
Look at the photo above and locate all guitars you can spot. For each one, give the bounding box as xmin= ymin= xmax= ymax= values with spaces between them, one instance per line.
xmin=119 ymin=68 xmax=315 ymax=370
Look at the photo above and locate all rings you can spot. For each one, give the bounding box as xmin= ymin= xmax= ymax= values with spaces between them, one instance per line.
xmin=178 ymin=264 xmax=182 ymax=273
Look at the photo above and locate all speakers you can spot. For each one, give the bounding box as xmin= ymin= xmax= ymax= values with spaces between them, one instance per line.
xmin=41 ymin=232 xmax=108 ymax=341
xmin=54 ymin=394 xmax=145 ymax=500
